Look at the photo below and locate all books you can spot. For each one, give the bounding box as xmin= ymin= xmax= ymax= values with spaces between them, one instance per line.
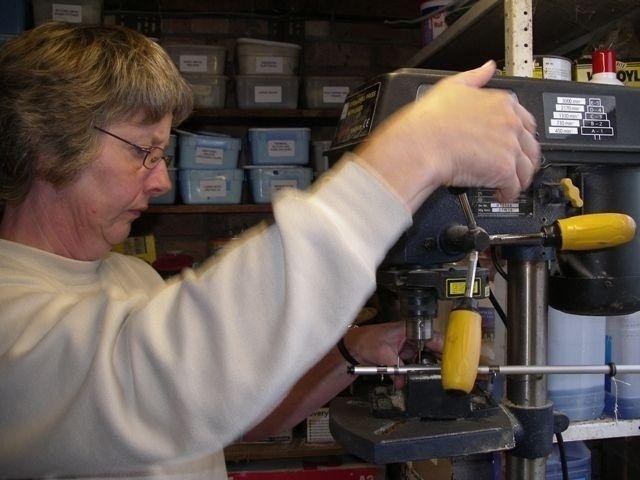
xmin=235 ymin=74 xmax=303 ymax=110
xmin=184 ymin=76 xmax=229 ymax=111
xmin=248 ymin=126 xmax=311 ymax=165
xmin=173 ymin=127 xmax=242 ymax=170
xmin=232 ymin=36 xmax=303 ymax=74
xmin=244 ymin=167 xmax=313 ymax=203
xmin=180 ymin=169 xmax=246 ymax=206
xmin=163 ymin=43 xmax=228 ymax=76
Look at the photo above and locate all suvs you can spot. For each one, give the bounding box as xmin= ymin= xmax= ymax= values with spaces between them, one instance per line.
xmin=494 ymin=262 xmax=605 ymax=419
xmin=605 ymin=312 xmax=640 ymax=421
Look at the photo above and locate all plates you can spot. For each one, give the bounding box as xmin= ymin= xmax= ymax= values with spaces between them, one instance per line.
xmin=149 ymin=117 xmax=343 ymax=204
xmin=99 ymin=7 xmax=387 ymax=108
xmin=225 ymin=457 xmax=407 ymax=479
xmin=390 ymin=0 xmax=476 ymax=70
xmin=111 ymin=213 xmax=277 ymax=285
xmin=414 ymin=1 xmax=639 ymax=446
xmin=409 ymin=436 xmax=639 ymax=479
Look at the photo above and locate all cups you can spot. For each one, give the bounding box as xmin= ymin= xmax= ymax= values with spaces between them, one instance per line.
xmin=94 ymin=125 xmax=175 ymax=170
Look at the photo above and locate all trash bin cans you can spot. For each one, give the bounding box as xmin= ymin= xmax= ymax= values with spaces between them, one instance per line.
xmin=336 ymin=322 xmax=360 ymax=364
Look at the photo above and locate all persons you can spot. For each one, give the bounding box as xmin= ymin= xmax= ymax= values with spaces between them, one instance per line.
xmin=0 ymin=21 xmax=543 ymax=478
